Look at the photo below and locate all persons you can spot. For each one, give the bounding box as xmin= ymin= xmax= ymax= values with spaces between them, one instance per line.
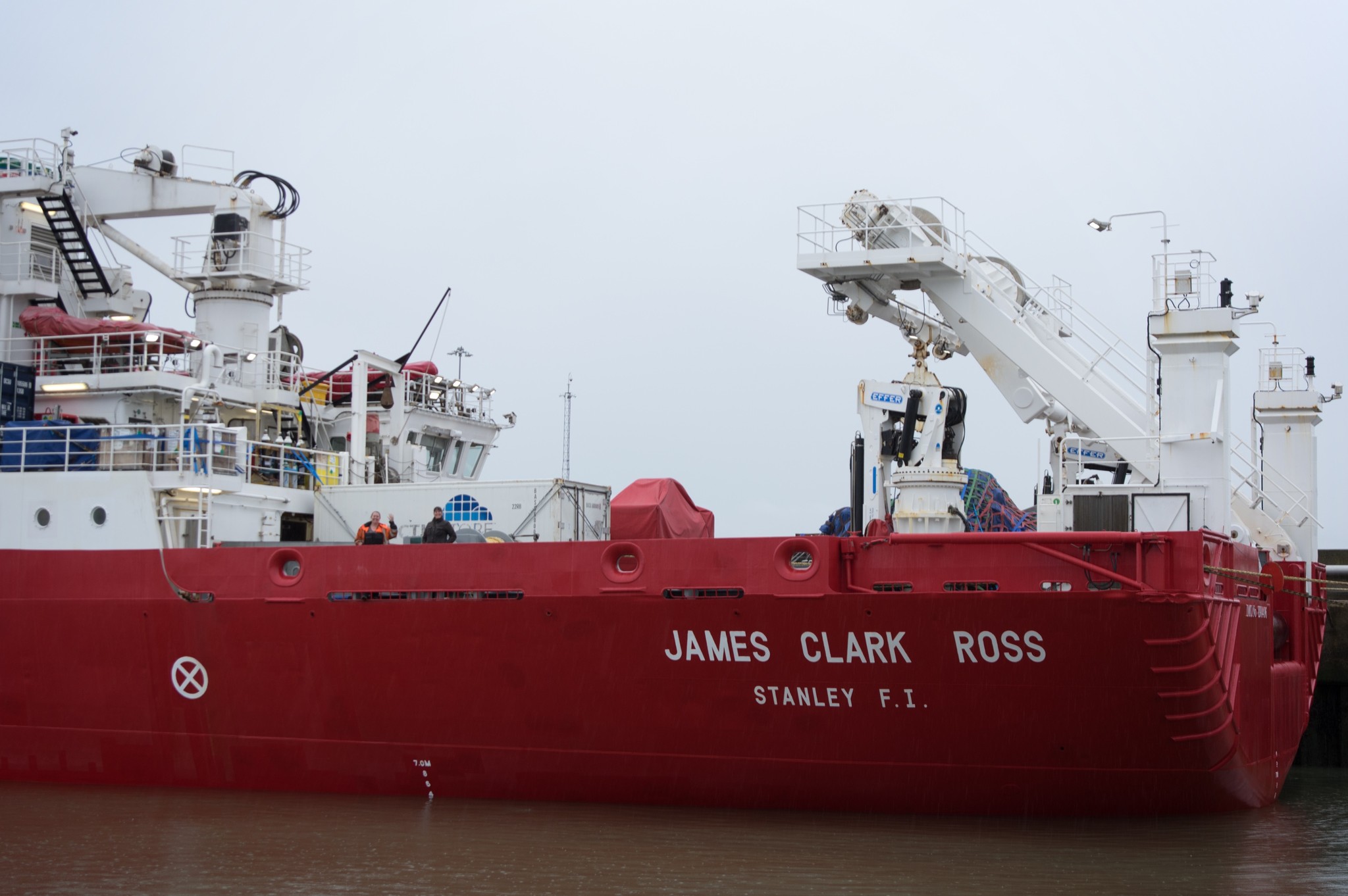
xmin=354 ymin=509 xmax=399 ymax=601
xmin=422 ymin=506 xmax=457 ymax=598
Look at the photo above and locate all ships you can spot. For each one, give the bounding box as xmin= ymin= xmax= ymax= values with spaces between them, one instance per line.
xmin=0 ymin=122 xmax=1346 ymax=817
